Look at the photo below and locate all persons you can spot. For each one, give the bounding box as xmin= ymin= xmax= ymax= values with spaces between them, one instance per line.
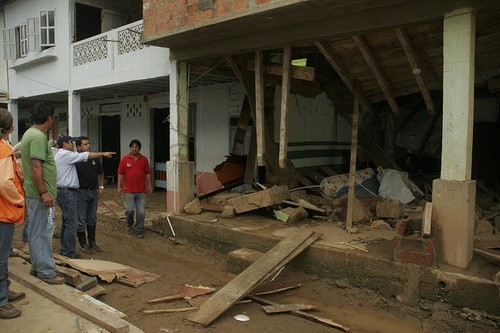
xmin=0 ymin=107 xmax=28 ymax=319
xmin=73 ymin=136 xmax=104 ymax=254
xmin=9 ymin=136 xmax=30 ymax=258
xmin=22 ymin=102 xmax=65 ymax=284
xmin=48 ymin=138 xmax=59 ymax=158
xmin=117 ymin=139 xmax=152 ymax=238
xmin=54 ymin=134 xmax=116 ymax=258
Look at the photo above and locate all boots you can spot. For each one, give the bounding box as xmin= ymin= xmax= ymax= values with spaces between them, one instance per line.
xmin=86 ymin=223 xmax=99 ymax=249
xmin=77 ymin=230 xmax=91 ymax=253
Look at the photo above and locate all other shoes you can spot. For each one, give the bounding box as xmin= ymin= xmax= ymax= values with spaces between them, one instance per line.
xmin=127 ymin=226 xmax=132 ymax=234
xmin=38 ymin=275 xmax=65 ymax=284
xmin=7 ymin=290 xmax=25 ymax=301
xmin=138 ymin=233 xmax=144 ymax=238
xmin=0 ymin=305 xmax=21 ymax=318
xmin=65 ymin=253 xmax=81 ymax=259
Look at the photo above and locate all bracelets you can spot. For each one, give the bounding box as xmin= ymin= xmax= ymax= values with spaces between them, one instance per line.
xmin=99 ymin=185 xmax=104 ymax=189
xmin=100 ymin=152 xmax=102 ymax=157
xmin=40 ymin=191 xmax=47 ymax=195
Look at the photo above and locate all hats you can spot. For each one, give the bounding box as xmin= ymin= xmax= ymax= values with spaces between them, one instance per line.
xmin=48 ymin=139 xmax=57 ymax=147
xmin=57 ymin=134 xmax=77 ymax=145
